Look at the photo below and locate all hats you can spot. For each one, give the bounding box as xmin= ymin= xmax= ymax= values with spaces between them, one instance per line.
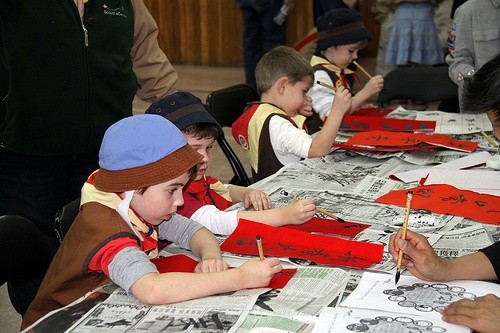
xmin=317 ymin=7 xmax=373 ymax=53
xmin=94 ymin=113 xmax=204 ymax=192
xmin=145 ymin=91 xmax=218 ymax=135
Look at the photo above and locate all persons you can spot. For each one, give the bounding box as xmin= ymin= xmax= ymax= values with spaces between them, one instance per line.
xmin=232 ymin=45 xmax=351 ymax=181
xmin=305 ymin=8 xmax=384 ymax=137
xmin=21 ymin=114 xmax=283 ymax=333
xmin=237 ymin=0 xmax=295 ymax=89
xmin=369 ymin=0 xmax=454 ymax=110
xmin=145 ymin=90 xmax=315 ymax=237
xmin=311 ymin=0 xmax=357 ymax=92
xmin=445 ymin=0 xmax=500 ymax=114
xmin=388 ymin=52 xmax=500 ymax=333
xmin=0 ymin=0 xmax=179 ymax=321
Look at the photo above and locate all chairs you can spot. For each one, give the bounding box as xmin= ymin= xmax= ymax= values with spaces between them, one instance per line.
xmin=0 ymin=214 xmax=54 ymax=292
xmin=206 ymin=84 xmax=260 ymax=185
xmin=377 ymin=66 xmax=458 ymax=107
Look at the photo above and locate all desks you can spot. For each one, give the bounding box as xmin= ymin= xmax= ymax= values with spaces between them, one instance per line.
xmin=16 ymin=109 xmax=500 ymax=333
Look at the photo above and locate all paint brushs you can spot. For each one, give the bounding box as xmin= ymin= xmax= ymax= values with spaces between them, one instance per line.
xmin=395 ymin=190 xmax=413 ymax=285
xmin=317 ymin=80 xmax=337 ymax=90
xmin=352 ymin=60 xmax=372 ymax=78
xmin=256 ymin=235 xmax=264 ymax=260
xmin=480 ymin=129 xmax=497 ymax=147
xmin=281 ymin=190 xmax=346 ymax=223
